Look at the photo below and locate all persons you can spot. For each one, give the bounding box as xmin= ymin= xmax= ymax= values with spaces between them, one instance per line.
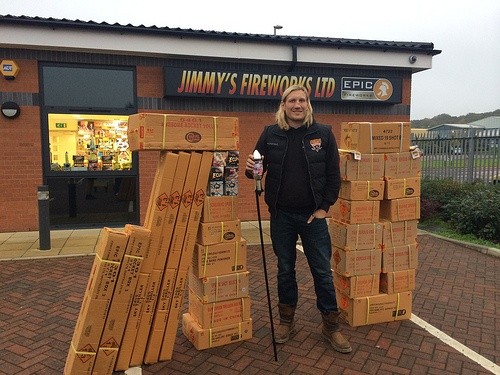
xmin=245 ymin=85 xmax=353 ymax=354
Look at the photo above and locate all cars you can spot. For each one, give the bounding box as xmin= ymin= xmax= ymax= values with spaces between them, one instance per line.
xmin=454 ymin=148 xmax=462 ymax=154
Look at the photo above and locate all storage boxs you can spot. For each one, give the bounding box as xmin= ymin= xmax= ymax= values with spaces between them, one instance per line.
xmin=327 ymin=122 xmax=421 ymax=328
xmin=128 ymin=113 xmax=240 ymax=151
xmin=63 ymin=152 xmax=253 ymax=375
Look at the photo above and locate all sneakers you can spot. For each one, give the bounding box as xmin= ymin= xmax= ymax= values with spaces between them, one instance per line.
xmin=274 ymin=304 xmax=297 ymax=343
xmin=322 ymin=312 xmax=352 ymax=352
